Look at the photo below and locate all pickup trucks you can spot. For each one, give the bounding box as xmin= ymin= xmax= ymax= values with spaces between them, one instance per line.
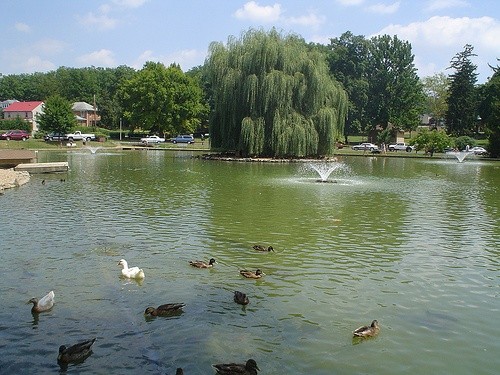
xmin=64 ymin=130 xmax=96 ymax=142
xmin=388 ymin=142 xmax=416 ymax=153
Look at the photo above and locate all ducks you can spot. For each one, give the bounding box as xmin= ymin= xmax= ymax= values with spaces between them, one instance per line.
xmin=239 ymin=269 xmax=265 ymax=279
xmin=117 ymin=259 xmax=144 ymax=279
xmin=352 ymin=320 xmax=381 ymax=338
xmin=189 ymin=258 xmax=219 ymax=268
xmin=233 ymin=290 xmax=249 ymax=305
xmin=210 ymin=359 xmax=261 ymax=375
xmin=252 ymin=244 xmax=274 ymax=252
xmin=26 ymin=290 xmax=55 ymax=312
xmin=144 ymin=302 xmax=186 ymax=317
xmin=57 ymin=337 xmax=96 ymax=362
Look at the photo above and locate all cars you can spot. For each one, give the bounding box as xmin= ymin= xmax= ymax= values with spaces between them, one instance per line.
xmin=353 ymin=143 xmax=378 ymax=151
xmin=141 ymin=136 xmax=165 ymax=144
xmin=44 ymin=131 xmax=66 ymax=141
xmin=0 ymin=130 xmax=30 ymax=141
xmin=463 ymin=147 xmax=488 ymax=155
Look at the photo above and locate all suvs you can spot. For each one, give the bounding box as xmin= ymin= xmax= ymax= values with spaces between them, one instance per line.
xmin=171 ymin=135 xmax=195 ymax=144
